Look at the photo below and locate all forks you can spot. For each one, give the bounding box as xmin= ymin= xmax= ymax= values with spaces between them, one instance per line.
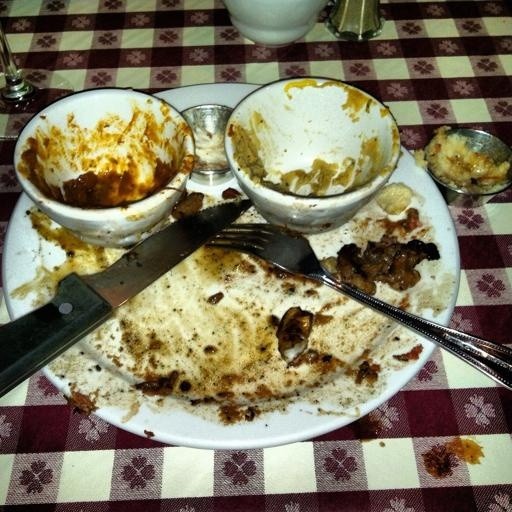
xmin=205 ymin=223 xmax=512 ymax=392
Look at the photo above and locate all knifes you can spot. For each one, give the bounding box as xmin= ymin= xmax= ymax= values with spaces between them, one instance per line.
xmin=0 ymin=199 xmax=254 ymax=402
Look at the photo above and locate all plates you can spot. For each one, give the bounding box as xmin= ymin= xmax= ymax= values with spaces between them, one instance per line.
xmin=2 ymin=82 xmax=461 ymax=450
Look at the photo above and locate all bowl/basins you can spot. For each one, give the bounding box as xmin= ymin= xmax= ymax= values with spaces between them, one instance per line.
xmin=222 ymin=77 xmax=403 ymax=236
xmin=420 ymin=127 xmax=512 ymax=210
xmin=13 ymin=88 xmax=197 ymax=249
xmin=180 ymin=104 xmax=235 ymax=187
